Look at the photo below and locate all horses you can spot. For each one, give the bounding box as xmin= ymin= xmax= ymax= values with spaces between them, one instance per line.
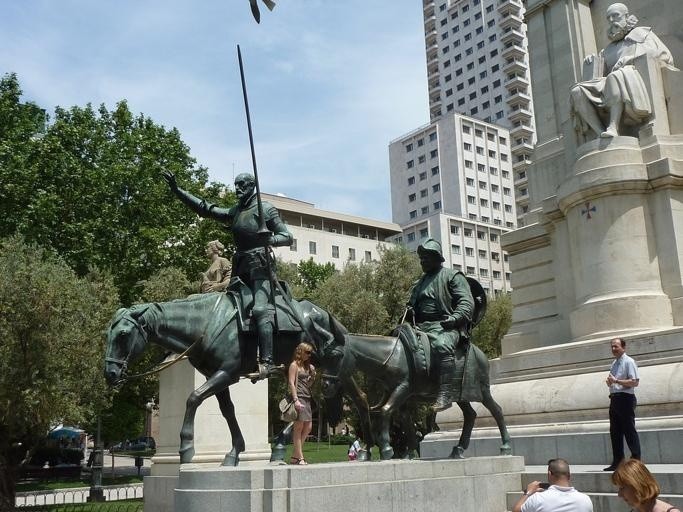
xmin=102 ymin=286 xmax=374 ymax=468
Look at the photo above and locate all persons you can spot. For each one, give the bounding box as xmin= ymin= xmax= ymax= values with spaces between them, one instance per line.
xmin=612 ymin=458 xmax=680 ymax=512
xmin=603 ymin=338 xmax=641 ymax=471
xmin=348 ymin=437 xmax=372 ymax=461
xmin=570 ymin=2 xmax=674 ymax=138
xmin=342 ymin=425 xmax=349 ymax=435
xmin=288 ymin=342 xmax=316 ymax=464
xmin=162 ymin=170 xmax=293 ymax=369
xmin=369 ymin=238 xmax=475 ymax=412
xmin=512 ymin=458 xmax=594 ymax=512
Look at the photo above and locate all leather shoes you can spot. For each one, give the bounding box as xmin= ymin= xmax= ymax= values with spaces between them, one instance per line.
xmin=603 ymin=465 xmax=615 ymax=471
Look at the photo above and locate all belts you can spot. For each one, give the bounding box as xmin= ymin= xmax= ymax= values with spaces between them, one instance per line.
xmin=609 ymin=392 xmax=626 ymax=398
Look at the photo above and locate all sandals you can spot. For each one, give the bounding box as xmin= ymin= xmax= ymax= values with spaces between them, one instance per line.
xmin=290 ymin=456 xmax=306 ymax=464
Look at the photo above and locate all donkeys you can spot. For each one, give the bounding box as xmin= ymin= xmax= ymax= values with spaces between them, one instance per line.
xmin=307 ymin=307 xmax=512 ymax=461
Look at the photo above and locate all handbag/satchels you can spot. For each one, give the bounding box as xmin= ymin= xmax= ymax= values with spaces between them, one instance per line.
xmin=311 ymin=398 xmax=318 ymax=410
xmin=279 ymin=398 xmax=298 ymax=422
xmin=348 ymin=449 xmax=357 ymax=459
